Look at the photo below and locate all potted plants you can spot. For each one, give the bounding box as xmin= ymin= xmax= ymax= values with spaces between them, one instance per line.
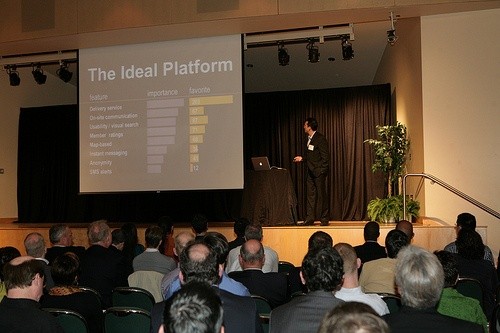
xmin=363 ymin=120 xmax=420 ymax=224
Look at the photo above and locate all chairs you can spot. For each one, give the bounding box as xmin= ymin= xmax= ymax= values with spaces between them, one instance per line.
xmin=111 ymin=287 xmax=156 ymax=314
xmin=41 ymin=307 xmax=90 ymax=333
xmin=364 ymin=291 xmax=403 ymax=314
xmin=249 ymin=293 xmax=272 ymax=314
xmin=78 ymin=287 xmax=106 ymax=316
xmin=276 ymin=261 xmax=306 ymax=294
xmin=101 ymin=306 xmax=154 ymax=333
xmin=452 ymin=276 xmax=486 ymax=308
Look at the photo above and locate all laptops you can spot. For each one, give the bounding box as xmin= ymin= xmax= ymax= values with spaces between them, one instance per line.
xmin=250 ymin=157 xmax=272 ymax=170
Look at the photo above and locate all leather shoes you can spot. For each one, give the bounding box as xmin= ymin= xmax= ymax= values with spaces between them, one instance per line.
xmin=320 ymin=221 xmax=328 ymax=226
xmin=299 ymin=219 xmax=313 ymax=226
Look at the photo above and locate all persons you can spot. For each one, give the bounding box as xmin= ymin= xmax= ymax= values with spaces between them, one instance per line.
xmin=0 ymin=213 xmax=500 ymax=333
xmin=293 ymin=117 xmax=330 ymax=226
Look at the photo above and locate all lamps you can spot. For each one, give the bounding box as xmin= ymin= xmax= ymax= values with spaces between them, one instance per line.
xmin=387 ymin=13 xmax=399 ymax=47
xmin=55 ymin=61 xmax=74 ymax=84
xmin=341 ymin=38 xmax=355 ymax=61
xmin=305 ymin=41 xmax=321 ymax=64
xmin=30 ymin=62 xmax=48 ymax=86
xmin=276 ymin=41 xmax=290 ymax=67
xmin=5 ymin=64 xmax=21 ymax=87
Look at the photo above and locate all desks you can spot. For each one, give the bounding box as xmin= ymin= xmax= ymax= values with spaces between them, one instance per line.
xmin=233 ymin=166 xmax=297 ymax=226
